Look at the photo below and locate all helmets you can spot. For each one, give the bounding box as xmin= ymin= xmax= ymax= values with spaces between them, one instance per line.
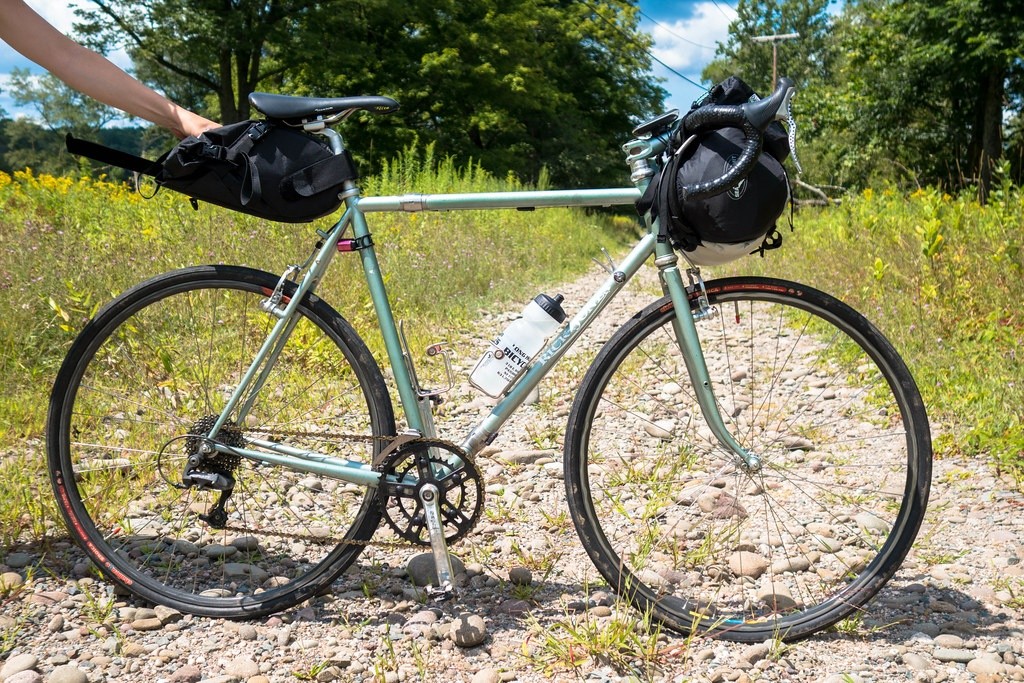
xmin=657 ymin=124 xmax=790 ymax=253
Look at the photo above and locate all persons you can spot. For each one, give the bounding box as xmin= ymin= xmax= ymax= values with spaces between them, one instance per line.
xmin=0 ymin=0 xmax=222 ymax=140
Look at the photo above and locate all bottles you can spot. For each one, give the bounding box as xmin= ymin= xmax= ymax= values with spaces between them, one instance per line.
xmin=469 ymin=291 xmax=568 ymax=398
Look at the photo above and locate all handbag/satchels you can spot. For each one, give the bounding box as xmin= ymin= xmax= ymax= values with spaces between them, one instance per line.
xmin=156 ymin=118 xmax=349 ymax=223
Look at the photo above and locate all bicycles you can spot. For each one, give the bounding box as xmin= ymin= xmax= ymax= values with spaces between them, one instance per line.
xmin=46 ymin=78 xmax=933 ymax=644
